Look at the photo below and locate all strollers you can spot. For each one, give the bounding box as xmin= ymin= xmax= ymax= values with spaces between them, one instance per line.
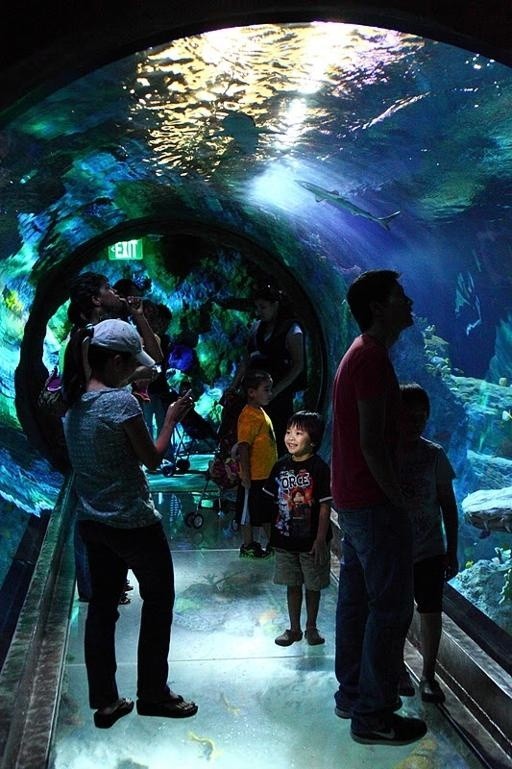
xmin=185 ymin=403 xmax=249 ymax=531
xmin=151 ymin=374 xmax=221 ymax=476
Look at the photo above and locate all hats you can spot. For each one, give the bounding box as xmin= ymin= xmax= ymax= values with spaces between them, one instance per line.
xmin=85 ymin=317 xmax=157 ymax=369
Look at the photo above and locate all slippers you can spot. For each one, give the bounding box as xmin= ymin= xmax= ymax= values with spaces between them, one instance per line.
xmin=94 ymin=696 xmax=136 ymax=730
xmin=137 ymin=693 xmax=200 ymax=720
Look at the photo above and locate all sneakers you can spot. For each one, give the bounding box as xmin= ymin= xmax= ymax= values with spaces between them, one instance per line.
xmin=349 ymin=712 xmax=428 ymax=748
xmin=334 ymin=686 xmax=403 ymax=720
xmin=396 ymin=669 xmax=417 ymax=698
xmin=418 ymin=676 xmax=447 ymax=704
xmin=239 ymin=540 xmax=275 ymax=558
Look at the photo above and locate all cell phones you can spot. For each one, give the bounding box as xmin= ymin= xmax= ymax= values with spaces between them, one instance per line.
xmin=182 ymin=389 xmax=192 ymax=399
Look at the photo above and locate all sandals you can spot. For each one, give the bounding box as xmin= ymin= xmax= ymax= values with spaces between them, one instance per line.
xmin=116 ymin=578 xmax=134 ymax=606
xmin=274 ymin=629 xmax=303 ymax=647
xmin=304 ymin=627 xmax=326 ymax=646
